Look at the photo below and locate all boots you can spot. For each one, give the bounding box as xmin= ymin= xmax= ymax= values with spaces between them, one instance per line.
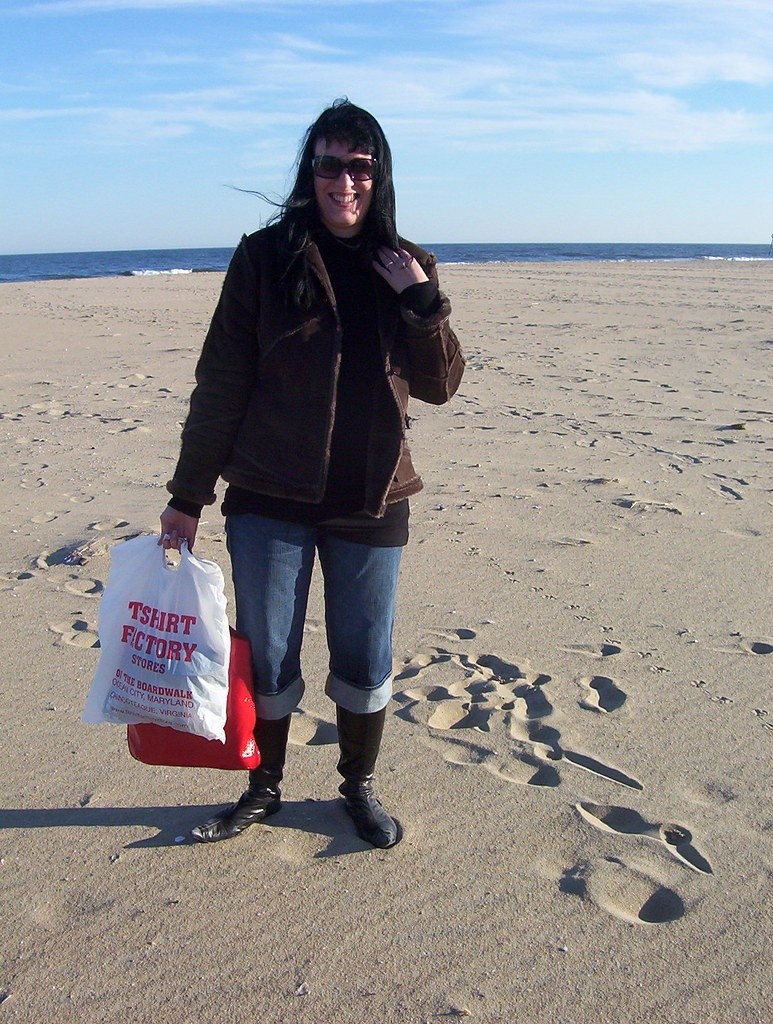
xmin=334 ymin=704 xmax=397 ymax=847
xmin=191 ymin=712 xmax=292 ymax=844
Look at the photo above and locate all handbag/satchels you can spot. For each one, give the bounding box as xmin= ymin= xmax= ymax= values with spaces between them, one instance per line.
xmin=124 ymin=621 xmax=260 ymax=770
xmin=79 ymin=536 xmax=230 ymax=745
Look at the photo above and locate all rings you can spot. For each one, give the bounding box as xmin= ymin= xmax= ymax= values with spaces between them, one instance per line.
xmin=385 ymin=262 xmax=393 ymax=267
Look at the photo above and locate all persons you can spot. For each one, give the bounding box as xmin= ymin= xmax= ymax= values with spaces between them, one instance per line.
xmin=160 ymin=100 xmax=468 ymax=848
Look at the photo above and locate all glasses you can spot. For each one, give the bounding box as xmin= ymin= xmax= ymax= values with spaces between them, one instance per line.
xmin=312 ymin=155 xmax=382 ymax=181
